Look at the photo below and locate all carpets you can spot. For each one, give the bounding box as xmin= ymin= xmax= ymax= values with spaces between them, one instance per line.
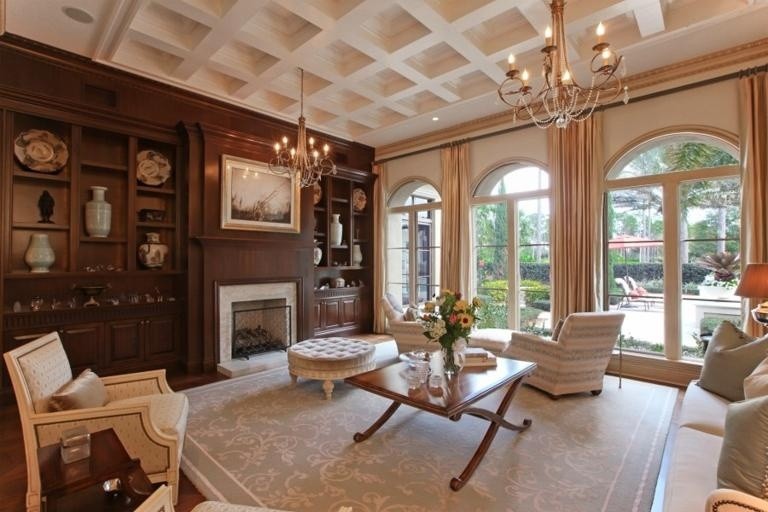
xmin=179 ymin=364 xmax=680 ymax=512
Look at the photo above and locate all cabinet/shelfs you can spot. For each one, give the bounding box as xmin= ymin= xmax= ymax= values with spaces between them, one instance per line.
xmin=308 ymin=166 xmax=371 ymax=297
xmin=1 ymin=99 xmax=182 ymax=319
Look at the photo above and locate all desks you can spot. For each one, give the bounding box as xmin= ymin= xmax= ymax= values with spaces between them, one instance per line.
xmin=344 ymin=346 xmax=537 ymax=491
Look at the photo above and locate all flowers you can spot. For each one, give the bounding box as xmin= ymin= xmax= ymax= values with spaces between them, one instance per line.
xmin=419 ymin=287 xmax=484 ymax=351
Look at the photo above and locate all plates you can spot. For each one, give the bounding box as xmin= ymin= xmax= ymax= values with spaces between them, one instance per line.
xmin=14 ymin=130 xmax=70 ymax=172
xmin=353 ymin=188 xmax=367 ymax=211
xmin=136 ymin=150 xmax=171 ymax=186
xmin=313 ymin=182 xmax=322 ymax=206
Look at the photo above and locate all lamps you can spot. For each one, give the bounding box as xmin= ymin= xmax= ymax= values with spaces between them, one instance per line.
xmin=495 ymin=0 xmax=631 ymax=130
xmin=265 ymin=72 xmax=339 ymax=187
xmin=733 ymin=260 xmax=767 ymax=311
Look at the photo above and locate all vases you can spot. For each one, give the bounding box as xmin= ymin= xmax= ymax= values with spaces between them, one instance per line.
xmin=309 ymin=212 xmax=365 ymax=269
xmin=24 ymin=234 xmax=58 ymax=275
xmin=436 ymin=344 xmax=466 ymax=375
xmin=137 ymin=232 xmax=170 ymax=270
xmin=83 ymin=186 xmax=115 ymax=239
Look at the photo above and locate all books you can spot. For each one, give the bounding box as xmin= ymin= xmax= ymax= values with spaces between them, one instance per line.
xmin=458 ymin=348 xmax=497 ymax=366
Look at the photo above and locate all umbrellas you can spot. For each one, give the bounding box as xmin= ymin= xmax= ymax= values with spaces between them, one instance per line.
xmin=608 ymin=234 xmax=663 ymax=286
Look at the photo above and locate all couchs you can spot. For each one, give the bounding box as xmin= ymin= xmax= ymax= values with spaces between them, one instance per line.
xmin=658 ymin=317 xmax=767 ymax=511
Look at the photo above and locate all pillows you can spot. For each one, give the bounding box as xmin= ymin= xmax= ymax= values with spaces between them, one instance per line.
xmin=551 ymin=318 xmax=564 ymax=343
xmin=402 ymin=298 xmax=422 ymax=320
xmin=692 ymin=318 xmax=767 ymax=499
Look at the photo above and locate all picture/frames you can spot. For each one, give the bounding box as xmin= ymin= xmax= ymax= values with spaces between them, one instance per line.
xmin=218 ymin=153 xmax=301 ymax=235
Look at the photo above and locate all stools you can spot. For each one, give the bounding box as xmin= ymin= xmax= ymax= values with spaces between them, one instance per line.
xmin=287 ymin=336 xmax=376 ymax=400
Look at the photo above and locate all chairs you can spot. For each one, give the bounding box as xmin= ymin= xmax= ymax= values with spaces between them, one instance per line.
xmin=380 ymin=291 xmax=513 ymax=365
xmin=502 ymin=312 xmax=627 ymax=396
xmin=613 ymin=274 xmax=664 ymax=313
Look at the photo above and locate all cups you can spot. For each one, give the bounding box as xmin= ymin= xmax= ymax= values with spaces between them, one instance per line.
xmin=407 ymin=366 xmax=441 ymax=390
